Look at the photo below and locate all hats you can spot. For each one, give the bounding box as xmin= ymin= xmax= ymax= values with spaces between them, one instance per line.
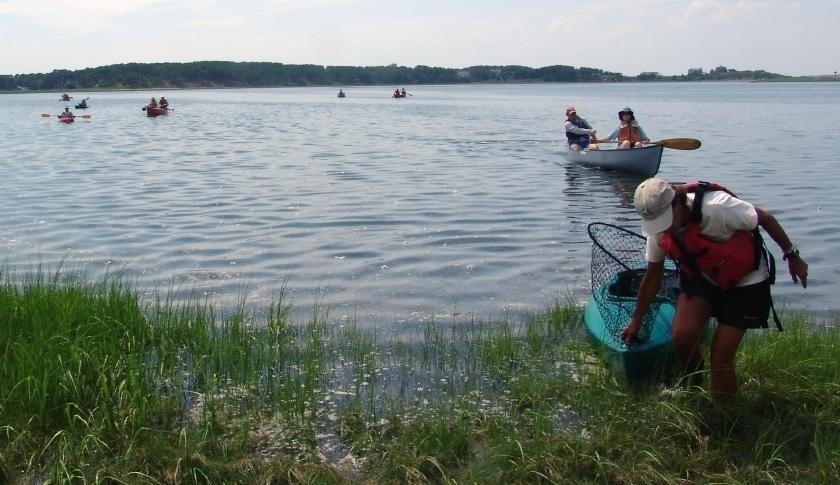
xmin=618 ymin=106 xmax=634 ymax=120
xmin=565 ymin=106 xmax=576 ymax=116
xmin=633 ymin=175 xmax=677 ymax=237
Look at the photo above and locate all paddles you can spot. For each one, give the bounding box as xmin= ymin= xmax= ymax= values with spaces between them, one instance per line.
xmin=406 ymin=92 xmax=413 ymax=96
xmin=590 ymin=137 xmax=701 ymax=150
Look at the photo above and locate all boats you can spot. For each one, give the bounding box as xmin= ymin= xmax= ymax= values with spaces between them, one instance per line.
xmin=585 ymin=257 xmax=680 ymax=380
xmin=63 ymin=94 xmax=70 ymax=101
xmin=566 ymin=145 xmax=663 ymax=177
xmin=394 ymin=91 xmax=406 ymax=97
xmin=58 ymin=114 xmax=73 ymax=122
xmin=147 ymin=104 xmax=167 ymax=117
xmin=338 ymin=94 xmax=345 ymax=97
xmin=75 ymin=99 xmax=88 ymax=108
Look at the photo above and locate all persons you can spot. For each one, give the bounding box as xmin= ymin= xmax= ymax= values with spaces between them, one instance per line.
xmin=339 ymin=89 xmax=343 ymax=95
xmin=564 ymin=107 xmax=599 ymax=153
xmin=158 ymin=95 xmax=169 ymax=110
xmin=402 ymin=88 xmax=406 ymax=95
xmin=81 ymin=98 xmax=87 ymax=107
xmin=622 ymin=178 xmax=808 ymax=403
xmin=595 ymin=108 xmax=651 ymax=152
xmin=149 ymin=97 xmax=157 ymax=108
xmin=64 ymin=95 xmax=69 ymax=98
xmin=61 ymin=106 xmax=73 ymax=117
xmin=395 ymin=89 xmax=400 ymax=94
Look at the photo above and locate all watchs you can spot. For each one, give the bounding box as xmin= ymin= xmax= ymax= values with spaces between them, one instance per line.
xmin=783 ymin=245 xmax=800 ymax=261
